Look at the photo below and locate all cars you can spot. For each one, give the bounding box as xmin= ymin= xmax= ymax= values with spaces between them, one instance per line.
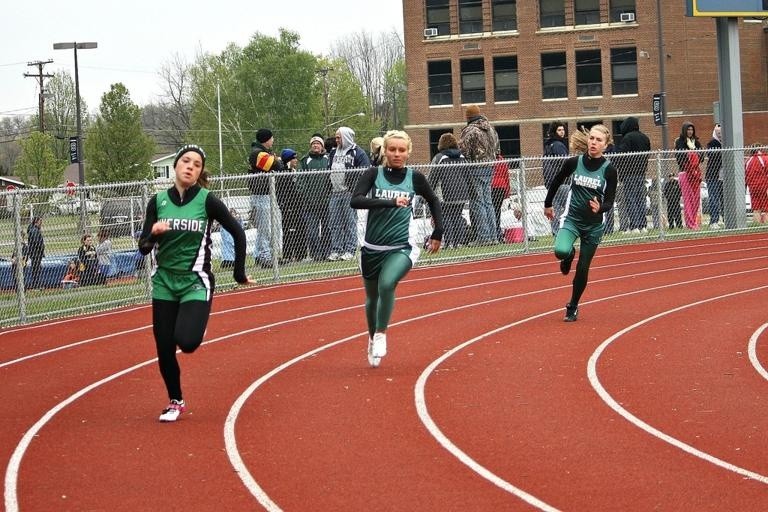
xmin=211 ymin=194 xmax=255 ymax=230
xmin=643 ymin=177 xmax=752 ymax=214
xmin=54 ymin=198 xmax=98 ymax=214
xmin=412 ymin=194 xmax=523 ymax=245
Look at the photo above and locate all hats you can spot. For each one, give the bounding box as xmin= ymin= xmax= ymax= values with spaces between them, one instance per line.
xmin=310 ymin=134 xmax=325 ymax=147
xmin=256 ymin=129 xmax=272 ymax=143
xmin=282 ymin=149 xmax=297 ymax=161
xmin=466 ymin=105 xmax=480 ymax=118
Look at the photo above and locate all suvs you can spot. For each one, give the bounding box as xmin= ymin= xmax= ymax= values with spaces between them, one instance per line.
xmin=99 ymin=196 xmax=148 ymax=240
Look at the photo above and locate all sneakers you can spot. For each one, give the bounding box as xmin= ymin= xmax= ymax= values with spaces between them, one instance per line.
xmin=368 ymin=333 xmax=387 ymax=367
xmin=564 ymin=303 xmax=578 ymax=321
xmin=469 ymin=240 xmax=498 ymax=246
xmin=255 ymin=253 xmax=354 ymax=267
xmin=560 ymin=248 xmax=575 ymax=274
xmin=711 ymin=223 xmax=720 ymax=230
xmin=159 ymin=399 xmax=185 ymax=421
xmin=621 ymin=228 xmax=647 ymax=234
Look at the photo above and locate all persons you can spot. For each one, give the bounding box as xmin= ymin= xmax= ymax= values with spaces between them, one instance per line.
xmin=135 ymin=143 xmax=254 ymax=422
xmin=220 ymin=127 xmax=371 ymax=269
xmin=602 ymin=117 xmax=767 ymax=234
xmin=422 ymin=105 xmax=510 ymax=251
xmin=10 ymin=216 xmax=45 ymax=293
xmin=541 ymin=122 xmax=624 ymax=323
xmin=62 ymin=215 xmax=153 ymax=287
xmin=347 ymin=129 xmax=449 ymax=371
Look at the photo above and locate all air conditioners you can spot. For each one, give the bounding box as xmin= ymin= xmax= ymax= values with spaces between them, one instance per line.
xmin=423 ymin=28 xmax=439 ymax=38
xmin=621 ymin=12 xmax=637 ymax=22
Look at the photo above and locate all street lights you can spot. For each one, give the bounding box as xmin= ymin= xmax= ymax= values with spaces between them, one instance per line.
xmin=326 ymin=112 xmax=364 ymax=139
